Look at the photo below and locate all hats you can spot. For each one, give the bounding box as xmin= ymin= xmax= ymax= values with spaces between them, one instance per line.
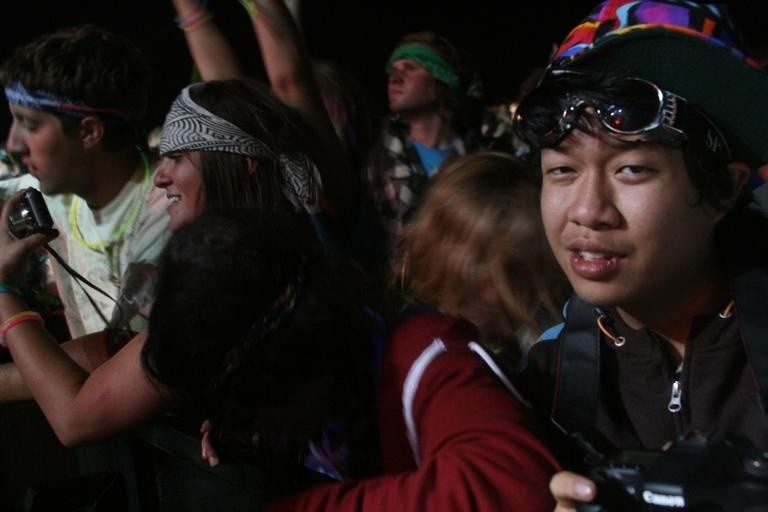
xmin=549 ymin=2 xmax=768 ymax=75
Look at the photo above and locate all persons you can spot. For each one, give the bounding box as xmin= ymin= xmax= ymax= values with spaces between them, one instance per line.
xmin=1 ymin=0 xmax=768 ymax=512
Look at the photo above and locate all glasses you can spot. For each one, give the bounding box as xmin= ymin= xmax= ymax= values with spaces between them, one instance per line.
xmin=511 ymin=68 xmax=726 ymax=155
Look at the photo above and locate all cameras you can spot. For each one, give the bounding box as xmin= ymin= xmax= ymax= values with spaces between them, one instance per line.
xmin=8 ymin=189 xmax=53 ymax=239
xmin=577 ymin=447 xmax=768 ymax=512
